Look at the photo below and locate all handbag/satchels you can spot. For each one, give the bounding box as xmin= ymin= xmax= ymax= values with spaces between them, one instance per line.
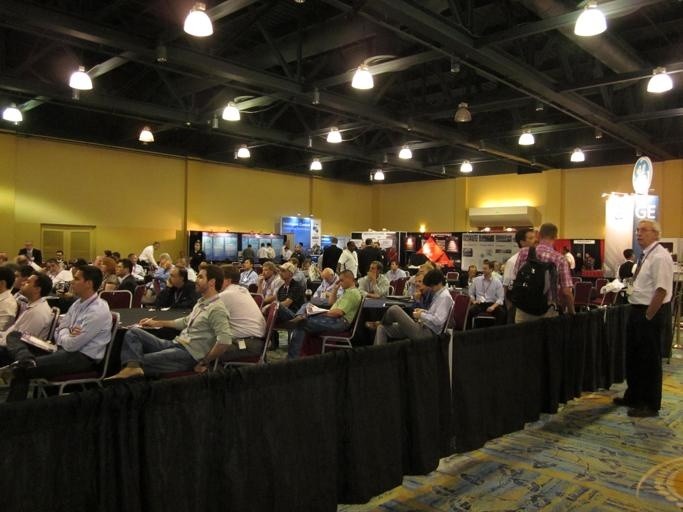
xmin=309 ymin=261 xmax=322 ymax=281
xmin=511 ymin=247 xmax=557 ymax=316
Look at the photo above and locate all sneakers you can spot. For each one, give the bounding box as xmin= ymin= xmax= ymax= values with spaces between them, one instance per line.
xmin=290 ymin=315 xmax=307 ymax=322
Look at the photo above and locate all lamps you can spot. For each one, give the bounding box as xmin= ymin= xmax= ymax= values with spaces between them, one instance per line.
xmin=518 ymin=128 xmax=534 ymax=145
xmin=139 ymin=126 xmax=154 ymax=142
xmin=574 ymin=1 xmax=607 ymax=36
xmin=221 ymin=100 xmax=241 ymax=122
xmin=374 ymin=169 xmax=385 ymax=180
xmin=455 ymin=101 xmax=471 ymax=122
xmin=183 ymin=1 xmax=213 ymax=37
xmin=2 ymin=102 xmax=22 ymax=123
xmin=460 ymin=159 xmax=473 ymax=173
xmin=351 ymin=18 xmax=374 ymax=90
xmin=647 ymin=66 xmax=673 ymax=93
xmin=570 ymin=148 xmax=585 ymax=162
xmin=237 ymin=144 xmax=250 ymax=158
xmin=69 ymin=64 xmax=92 ymax=91
xmin=399 ymin=143 xmax=412 ymax=160
xmin=327 ymin=126 xmax=342 ymax=143
xmin=310 ymin=157 xmax=322 ymax=170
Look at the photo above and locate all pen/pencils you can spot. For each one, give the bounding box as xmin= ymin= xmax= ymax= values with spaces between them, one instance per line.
xmin=138 ymin=315 xmax=157 ymax=327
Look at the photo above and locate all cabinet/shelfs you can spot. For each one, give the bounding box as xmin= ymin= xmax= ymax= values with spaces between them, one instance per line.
xmin=279 ymin=215 xmax=321 ymax=256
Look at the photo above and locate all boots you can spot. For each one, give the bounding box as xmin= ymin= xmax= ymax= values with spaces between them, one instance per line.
xmin=266 ymin=332 xmax=278 ymax=351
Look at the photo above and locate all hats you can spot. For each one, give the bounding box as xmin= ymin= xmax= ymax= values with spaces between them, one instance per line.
xmin=278 ymin=262 xmax=295 ymax=274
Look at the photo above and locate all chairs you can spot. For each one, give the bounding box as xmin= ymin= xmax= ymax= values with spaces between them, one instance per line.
xmin=0 ymin=270 xmax=622 ymax=400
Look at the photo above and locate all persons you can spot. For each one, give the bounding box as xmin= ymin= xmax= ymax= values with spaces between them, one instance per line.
xmin=219 ymin=267 xmax=266 ymax=361
xmin=1 ymin=240 xmax=232 ymax=396
xmin=615 ymin=249 xmax=637 ymax=305
xmin=612 ymin=217 xmax=673 ymax=418
xmin=288 ymin=238 xmax=389 ymax=356
xmin=503 ymin=223 xmax=576 ymax=325
xmin=237 ymin=241 xmax=316 ymax=351
xmin=460 ymin=259 xmax=507 ymax=330
xmin=574 ymin=252 xmax=594 ymax=272
xmin=373 ymin=260 xmax=453 ymax=346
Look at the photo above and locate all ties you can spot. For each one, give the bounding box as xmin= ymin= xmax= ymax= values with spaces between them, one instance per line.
xmin=631 ymin=254 xmax=644 ymax=280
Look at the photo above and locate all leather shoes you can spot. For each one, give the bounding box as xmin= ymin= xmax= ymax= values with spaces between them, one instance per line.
xmin=365 ymin=322 xmax=376 ymax=330
xmin=103 ymin=368 xmax=144 ymax=384
xmin=613 ymin=397 xmax=656 ymax=416
xmin=0 ymin=360 xmax=36 ymax=378
